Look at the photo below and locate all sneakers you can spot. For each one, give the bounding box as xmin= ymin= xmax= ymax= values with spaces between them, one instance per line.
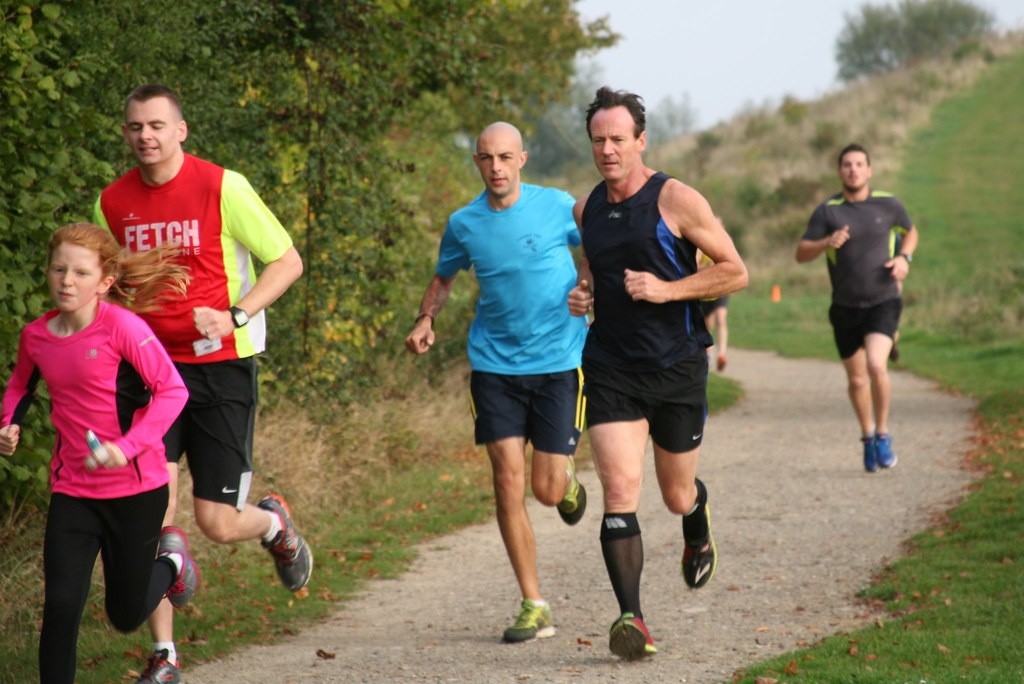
xmin=681 ymin=503 xmax=717 ymax=589
xmin=158 ymin=525 xmax=201 ymax=609
xmin=503 ymin=599 xmax=556 ymax=643
xmin=861 ymin=429 xmax=897 ymax=473
xmin=134 ymin=649 xmax=182 ymax=684
xmin=257 ymin=493 xmax=312 ymax=591
xmin=609 ymin=612 xmax=657 ymax=662
xmin=556 ymin=454 xmax=587 ymax=526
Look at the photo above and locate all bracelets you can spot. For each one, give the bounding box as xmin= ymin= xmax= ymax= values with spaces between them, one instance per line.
xmin=412 ymin=312 xmax=437 ymax=329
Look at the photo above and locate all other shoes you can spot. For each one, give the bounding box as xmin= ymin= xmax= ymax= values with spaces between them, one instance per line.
xmin=891 ymin=343 xmax=899 ymax=362
xmin=718 ymin=355 xmax=727 ymax=372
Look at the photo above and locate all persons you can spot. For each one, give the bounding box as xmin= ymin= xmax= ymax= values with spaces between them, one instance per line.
xmin=564 ymin=85 xmax=750 ymax=660
xmin=85 ymin=84 xmax=315 ymax=683
xmin=692 ymin=218 xmax=730 ymax=369
xmin=795 ymin=143 xmax=920 ymax=474
xmin=401 ymin=120 xmax=598 ymax=644
xmin=0 ymin=221 xmax=201 ymax=684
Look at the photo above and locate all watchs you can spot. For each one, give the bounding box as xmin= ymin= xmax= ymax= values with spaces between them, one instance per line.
xmin=899 ymin=253 xmax=912 ymax=264
xmin=228 ymin=306 xmax=250 ymax=330
